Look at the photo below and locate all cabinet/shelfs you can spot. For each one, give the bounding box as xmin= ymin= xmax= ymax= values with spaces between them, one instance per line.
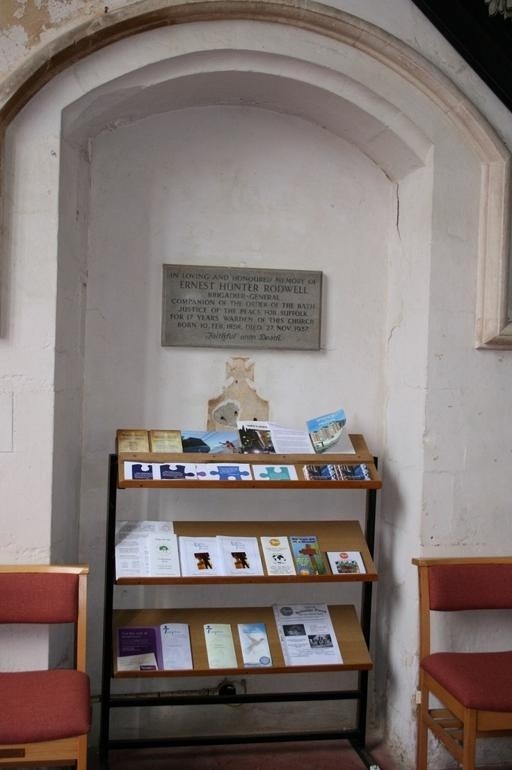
xmin=100 ymin=434 xmax=382 ymax=770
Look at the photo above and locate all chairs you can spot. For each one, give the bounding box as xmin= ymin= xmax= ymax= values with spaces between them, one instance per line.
xmin=0 ymin=564 xmax=90 ymax=770
xmin=412 ymin=556 xmax=511 ymax=769
